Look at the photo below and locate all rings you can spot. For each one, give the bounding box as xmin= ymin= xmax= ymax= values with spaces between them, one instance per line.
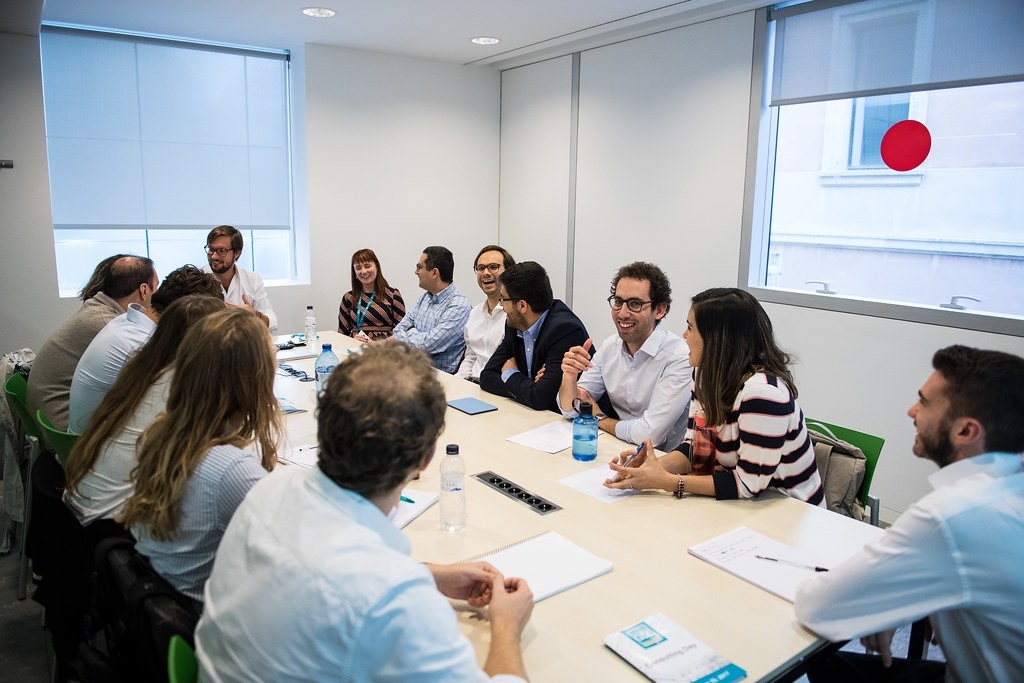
xmin=625 ymin=479 xmax=633 ymax=490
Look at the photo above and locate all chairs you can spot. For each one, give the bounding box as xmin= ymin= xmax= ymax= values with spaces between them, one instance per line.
xmin=802 ymin=417 xmax=884 ymax=526
xmin=0 ymin=361 xmax=204 ymax=683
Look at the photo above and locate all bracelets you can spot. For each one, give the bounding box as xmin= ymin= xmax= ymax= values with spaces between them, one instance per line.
xmin=674 ymin=472 xmax=684 ymax=499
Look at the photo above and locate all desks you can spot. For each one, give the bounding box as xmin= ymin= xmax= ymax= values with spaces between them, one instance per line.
xmin=220 ymin=331 xmax=931 ymax=683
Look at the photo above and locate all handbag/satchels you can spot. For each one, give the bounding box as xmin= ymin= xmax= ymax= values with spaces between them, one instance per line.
xmin=805 ymin=422 xmax=867 ymax=516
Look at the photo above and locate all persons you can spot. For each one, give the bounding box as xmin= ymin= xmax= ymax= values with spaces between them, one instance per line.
xmin=194 ymin=339 xmax=534 ymax=683
xmin=602 ymin=288 xmax=827 ymax=508
xmin=25 ymin=254 xmax=287 ymax=604
xmin=453 ymin=245 xmax=516 ymax=385
xmin=556 ymin=261 xmax=692 ymax=452
xmin=201 ymin=225 xmax=279 ymax=336
xmin=794 ymin=344 xmax=1024 ymax=683
xmin=392 ymin=246 xmax=472 ymax=375
xmin=337 ymin=249 xmax=406 ymax=345
xmin=480 ymin=261 xmax=618 ymax=420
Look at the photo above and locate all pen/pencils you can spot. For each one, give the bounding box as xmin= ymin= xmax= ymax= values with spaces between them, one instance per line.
xmin=299 ymin=446 xmax=318 ymax=452
xmin=610 ymin=443 xmax=644 ymax=481
xmin=756 ymin=555 xmax=828 ymax=572
xmin=400 ymin=495 xmax=414 ymax=504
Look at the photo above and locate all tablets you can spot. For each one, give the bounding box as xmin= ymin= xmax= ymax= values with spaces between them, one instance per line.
xmin=446 ymin=397 xmax=498 ymax=415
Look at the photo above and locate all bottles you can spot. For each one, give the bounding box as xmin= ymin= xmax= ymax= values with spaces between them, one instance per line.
xmin=572 ymin=397 xmax=598 ymax=461
xmin=440 ymin=445 xmax=465 ymax=530
xmin=315 ymin=344 xmax=339 ymax=391
xmin=687 ymin=409 xmax=717 ymax=475
xmin=305 ymin=306 xmax=317 ymax=341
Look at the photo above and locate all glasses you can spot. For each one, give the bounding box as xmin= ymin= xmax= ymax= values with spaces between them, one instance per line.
xmin=475 ymin=262 xmax=505 ymax=274
xmin=204 ymin=243 xmax=233 ymax=257
xmin=498 ymin=296 xmax=528 ymax=306
xmin=607 ymin=294 xmax=656 ymax=313
xmin=416 ymin=263 xmax=427 ymax=270
